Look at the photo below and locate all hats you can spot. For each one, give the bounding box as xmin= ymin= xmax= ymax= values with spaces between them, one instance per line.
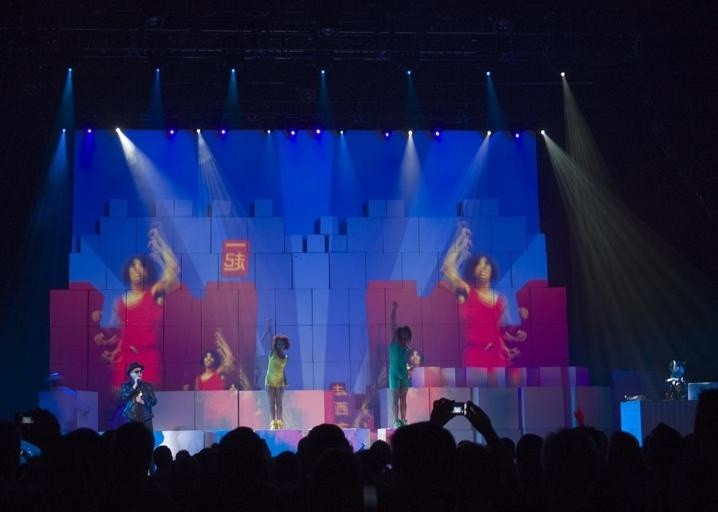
xmin=128 ymin=363 xmax=144 ymax=375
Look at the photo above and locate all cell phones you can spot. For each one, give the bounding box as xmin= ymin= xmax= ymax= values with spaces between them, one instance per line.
xmin=18 ymin=440 xmax=42 ymax=466
xmin=452 ymin=402 xmax=467 ymax=415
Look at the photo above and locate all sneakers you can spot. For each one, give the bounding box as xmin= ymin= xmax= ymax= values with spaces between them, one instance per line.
xmin=393 ymin=418 xmax=407 ymax=428
xmin=272 ymin=420 xmax=284 ymax=430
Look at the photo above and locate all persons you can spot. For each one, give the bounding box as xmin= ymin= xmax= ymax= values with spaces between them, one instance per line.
xmin=439 ymin=221 xmax=529 ymax=387
xmin=407 ymin=350 xmax=431 ymax=385
xmin=119 ymin=361 xmax=155 ymax=432
xmin=92 ymin=225 xmax=182 ymax=388
xmin=388 ymin=299 xmax=413 ymax=426
xmin=12 ymin=399 xmax=718 ymax=511
xmin=181 ymin=327 xmax=239 ymax=391
xmin=261 ymin=318 xmax=294 ymax=429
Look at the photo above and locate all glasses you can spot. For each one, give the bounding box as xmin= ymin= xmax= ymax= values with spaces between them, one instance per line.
xmin=133 ymin=370 xmax=142 ymax=373
